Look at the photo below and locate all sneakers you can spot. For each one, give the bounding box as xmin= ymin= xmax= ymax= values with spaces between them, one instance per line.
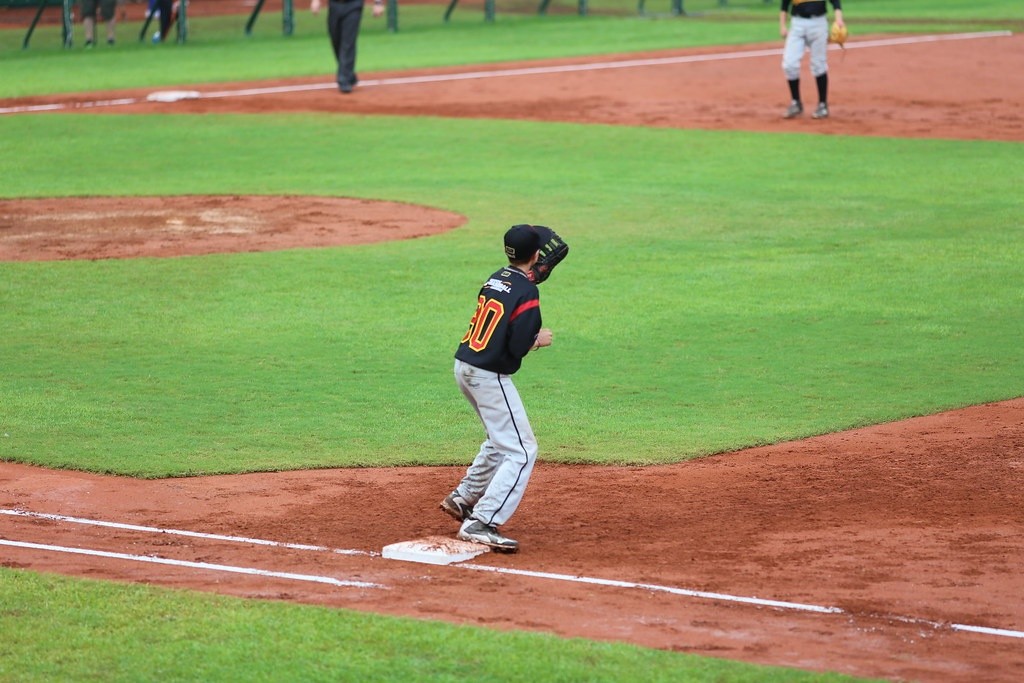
xmin=811 ymin=102 xmax=828 ymax=118
xmin=783 ymin=99 xmax=802 ymax=118
xmin=439 ymin=489 xmax=474 ymax=523
xmin=456 ymin=518 xmax=519 ymax=553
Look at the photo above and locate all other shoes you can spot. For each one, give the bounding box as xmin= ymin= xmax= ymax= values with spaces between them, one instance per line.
xmin=340 ymin=81 xmax=352 ymax=92
xmin=352 ymin=76 xmax=357 ymax=85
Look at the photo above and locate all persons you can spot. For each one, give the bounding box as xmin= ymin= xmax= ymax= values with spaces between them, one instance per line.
xmin=79 ymin=0 xmax=116 ymax=49
xmin=310 ymin=0 xmax=383 ymax=92
xmin=779 ymin=0 xmax=848 ymax=118
xmin=145 ymin=0 xmax=189 ymax=43
xmin=440 ymin=224 xmax=569 ymax=549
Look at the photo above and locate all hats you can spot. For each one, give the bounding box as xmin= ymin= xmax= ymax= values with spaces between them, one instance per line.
xmin=504 ymin=224 xmax=552 ymax=259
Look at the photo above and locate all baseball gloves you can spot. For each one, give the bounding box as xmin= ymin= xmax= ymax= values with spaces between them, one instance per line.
xmin=828 ymin=22 xmax=849 ymax=43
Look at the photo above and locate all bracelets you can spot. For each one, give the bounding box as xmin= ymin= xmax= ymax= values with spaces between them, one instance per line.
xmin=532 ymin=339 xmax=540 ymax=351
xmin=376 ymin=2 xmax=382 ymax=5
